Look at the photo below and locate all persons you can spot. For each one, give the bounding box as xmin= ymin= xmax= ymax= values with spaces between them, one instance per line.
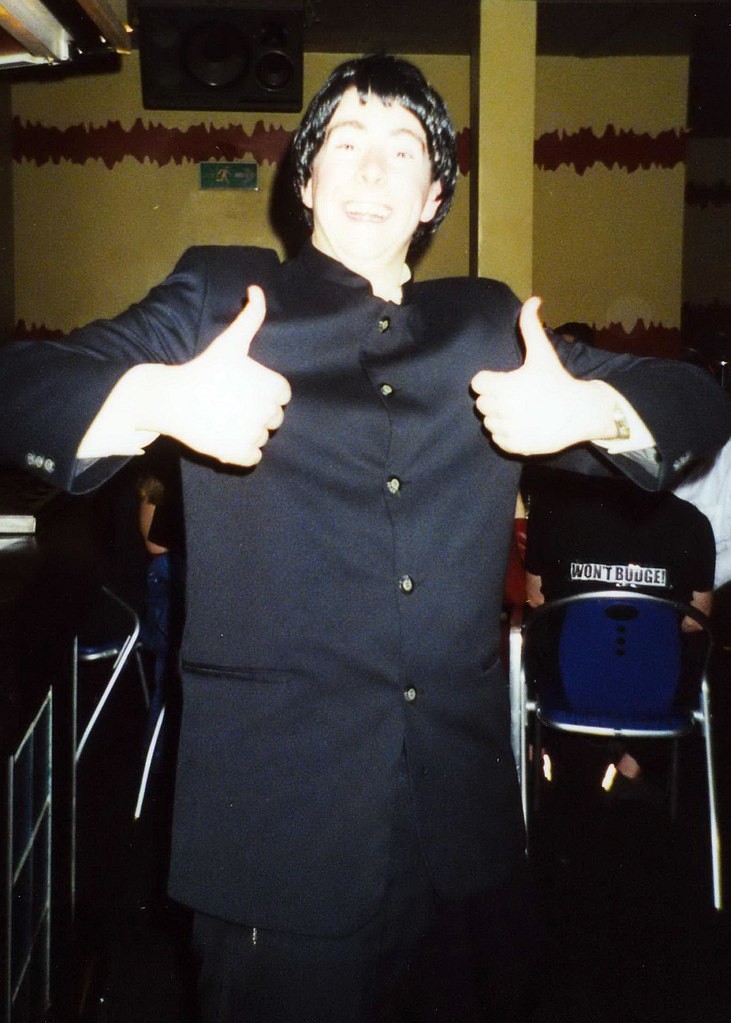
xmin=0 ymin=52 xmax=731 ymax=1023
xmin=517 ymin=324 xmax=731 ymax=801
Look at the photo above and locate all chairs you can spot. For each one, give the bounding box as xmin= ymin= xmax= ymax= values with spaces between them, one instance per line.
xmin=21 ymin=521 xmax=180 ymax=819
xmin=503 ymin=590 xmax=727 ymax=914
